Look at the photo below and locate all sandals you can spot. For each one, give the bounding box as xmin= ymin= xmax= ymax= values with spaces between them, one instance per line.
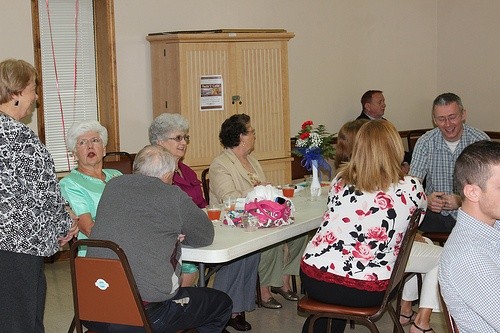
xmin=397 ymin=309 xmax=418 ymax=327
xmin=408 ymin=320 xmax=436 ymax=333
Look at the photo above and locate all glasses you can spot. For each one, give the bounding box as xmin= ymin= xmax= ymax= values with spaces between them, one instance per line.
xmin=245 ymin=128 xmax=256 ymax=134
xmin=77 ymin=138 xmax=103 ymax=146
xmin=168 ymin=134 xmax=189 ymax=143
xmin=434 ymin=108 xmax=463 ymax=122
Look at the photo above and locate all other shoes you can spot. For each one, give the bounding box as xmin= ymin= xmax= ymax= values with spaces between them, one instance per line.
xmin=229 ymin=314 xmax=251 ymax=331
xmin=257 ymin=294 xmax=280 ymax=310
xmin=271 ymin=283 xmax=299 ymax=302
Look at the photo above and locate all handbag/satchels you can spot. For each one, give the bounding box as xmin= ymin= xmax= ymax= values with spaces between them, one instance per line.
xmin=244 ymin=197 xmax=294 ymax=229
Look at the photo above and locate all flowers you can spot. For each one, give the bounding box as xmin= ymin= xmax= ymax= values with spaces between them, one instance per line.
xmin=289 ymin=120 xmax=338 ymax=161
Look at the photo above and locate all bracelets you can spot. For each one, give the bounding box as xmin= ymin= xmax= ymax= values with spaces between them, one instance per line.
xmin=72 ymin=217 xmax=80 ymax=220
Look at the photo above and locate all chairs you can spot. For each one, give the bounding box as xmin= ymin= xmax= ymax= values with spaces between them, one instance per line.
xmin=297 ymin=207 xmax=459 ymax=333
xmin=70 ymin=240 xmax=196 ymax=333
xmin=102 ymin=151 xmax=134 ymax=175
xmin=202 ymin=168 xmax=297 ymax=307
xmin=408 ymin=130 xmax=425 ymax=151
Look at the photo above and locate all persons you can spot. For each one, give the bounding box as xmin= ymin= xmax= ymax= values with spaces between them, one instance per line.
xmin=208 ymin=113 xmax=309 ymax=309
xmin=437 ymin=139 xmax=500 ymax=333
xmin=334 ymin=118 xmax=443 ymax=333
xmin=354 ymin=90 xmax=411 ymax=177
xmin=58 ymin=118 xmax=199 ymax=287
xmin=0 ymin=60 xmax=79 ymax=333
xmin=83 ymin=143 xmax=233 ymax=333
xmin=148 ymin=112 xmax=261 ymax=333
xmin=298 ymin=118 xmax=428 ymax=333
xmin=408 ymin=92 xmax=491 ymax=233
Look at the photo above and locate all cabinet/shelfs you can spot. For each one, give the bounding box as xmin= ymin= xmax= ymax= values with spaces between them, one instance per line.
xmin=147 ymin=32 xmax=295 ymax=199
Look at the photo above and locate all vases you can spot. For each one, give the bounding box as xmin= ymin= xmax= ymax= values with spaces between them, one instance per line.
xmin=310 ymin=160 xmax=321 ymax=196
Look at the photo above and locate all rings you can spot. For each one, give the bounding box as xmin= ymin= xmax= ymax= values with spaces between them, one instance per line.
xmin=444 ymin=202 xmax=446 ymax=207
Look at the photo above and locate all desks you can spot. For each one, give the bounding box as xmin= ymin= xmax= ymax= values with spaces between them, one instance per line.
xmin=181 ymin=181 xmax=332 ymax=289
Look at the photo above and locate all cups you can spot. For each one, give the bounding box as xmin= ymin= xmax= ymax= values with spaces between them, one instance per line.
xmin=206 ymin=204 xmax=224 ymax=223
xmin=304 ymin=175 xmax=313 ymax=190
xmin=223 ymin=196 xmax=237 ymax=214
xmin=240 ymin=215 xmax=259 ymax=231
xmin=281 ymin=183 xmax=295 ymax=198
